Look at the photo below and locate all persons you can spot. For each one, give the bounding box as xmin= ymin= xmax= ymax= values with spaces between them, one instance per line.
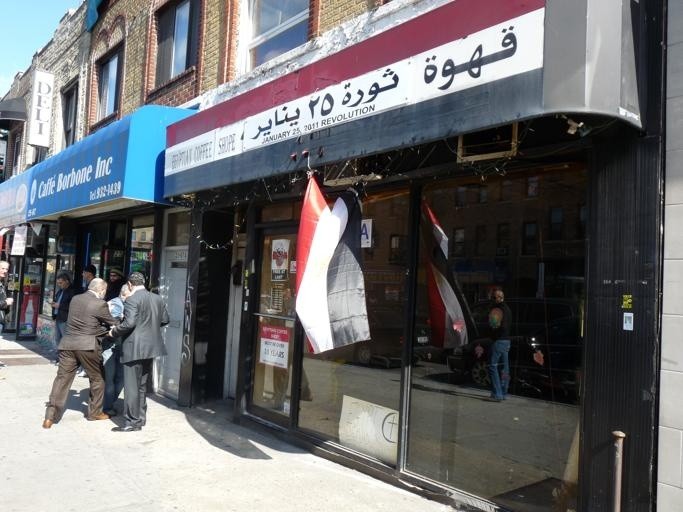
xmin=484 ymin=289 xmax=511 ymax=401
xmin=101 ymin=283 xmax=131 ymax=417
xmin=81 ymin=265 xmax=94 ymax=284
xmin=103 ymin=267 xmax=123 ymax=300
xmin=47 ymin=272 xmax=74 ymax=365
xmin=42 ymin=276 xmax=125 ymax=429
xmin=0 ymin=260 xmax=9 ymax=367
xmin=104 ymin=271 xmax=168 ymax=432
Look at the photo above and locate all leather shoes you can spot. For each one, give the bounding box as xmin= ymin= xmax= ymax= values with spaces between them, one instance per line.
xmin=111 ymin=424 xmax=143 ymax=433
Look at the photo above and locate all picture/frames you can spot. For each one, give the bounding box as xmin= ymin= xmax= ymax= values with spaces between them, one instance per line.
xmin=452 ymin=200 xmax=588 ymax=258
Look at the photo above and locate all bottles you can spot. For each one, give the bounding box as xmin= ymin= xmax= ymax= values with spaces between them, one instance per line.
xmin=24 ymin=295 xmax=35 ymax=336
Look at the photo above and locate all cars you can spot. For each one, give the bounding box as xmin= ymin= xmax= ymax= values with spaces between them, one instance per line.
xmin=447 ymin=335 xmax=580 ymax=401
xmin=306 ymin=306 xmax=419 ymax=369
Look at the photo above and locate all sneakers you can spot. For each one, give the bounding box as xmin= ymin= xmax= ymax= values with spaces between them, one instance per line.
xmin=486 ymin=396 xmax=500 ymax=401
xmin=87 ymin=411 xmax=111 ymax=421
xmin=42 ymin=417 xmax=54 ymax=429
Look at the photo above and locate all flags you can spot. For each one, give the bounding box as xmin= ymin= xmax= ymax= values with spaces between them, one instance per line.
xmin=291 ymin=176 xmax=372 ymax=354
xmin=418 ymin=200 xmax=479 ymax=355
